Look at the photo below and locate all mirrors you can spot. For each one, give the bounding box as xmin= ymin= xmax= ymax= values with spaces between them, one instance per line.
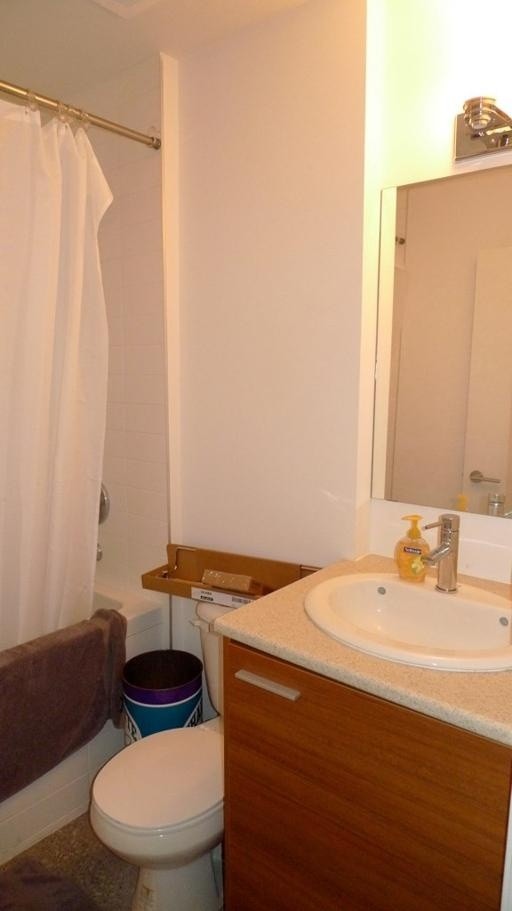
xmin=371 ymin=152 xmax=511 ymax=519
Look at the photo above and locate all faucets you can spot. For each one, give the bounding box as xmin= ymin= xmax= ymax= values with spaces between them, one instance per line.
xmin=421 ymin=515 xmax=459 ymax=594
xmin=487 ymin=493 xmax=512 ymax=519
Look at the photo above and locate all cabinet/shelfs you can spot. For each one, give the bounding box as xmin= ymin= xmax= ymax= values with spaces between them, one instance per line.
xmin=219 ymin=634 xmax=510 ymax=911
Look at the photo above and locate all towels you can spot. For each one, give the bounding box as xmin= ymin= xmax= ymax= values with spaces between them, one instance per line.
xmin=0 ymin=608 xmax=130 ymax=806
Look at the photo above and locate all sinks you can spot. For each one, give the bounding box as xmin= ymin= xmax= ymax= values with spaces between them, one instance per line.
xmin=304 ymin=573 xmax=512 ymax=673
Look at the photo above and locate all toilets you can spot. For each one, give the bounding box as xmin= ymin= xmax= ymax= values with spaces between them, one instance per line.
xmin=90 ymin=601 xmax=238 ymax=911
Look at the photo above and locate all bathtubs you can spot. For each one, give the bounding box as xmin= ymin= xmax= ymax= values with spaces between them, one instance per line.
xmin=1 ymin=583 xmax=161 ymax=866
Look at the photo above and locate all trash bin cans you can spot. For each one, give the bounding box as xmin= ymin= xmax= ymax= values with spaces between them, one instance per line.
xmin=119 ymin=650 xmax=203 ymax=747
